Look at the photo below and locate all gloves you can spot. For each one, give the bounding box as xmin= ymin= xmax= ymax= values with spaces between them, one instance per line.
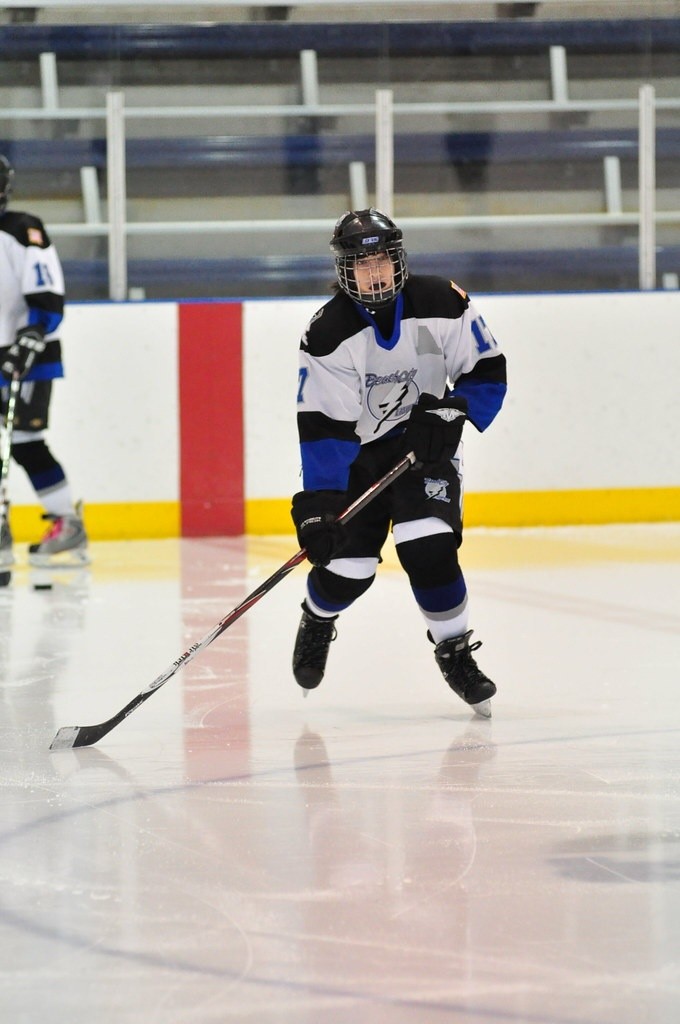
xmin=1 ymin=325 xmax=46 ymax=382
xmin=405 ymin=392 xmax=467 ymax=463
xmin=291 ymin=490 xmax=350 ymax=568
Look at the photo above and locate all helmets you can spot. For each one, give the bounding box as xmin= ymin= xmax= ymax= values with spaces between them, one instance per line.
xmin=0 ymin=155 xmax=14 ymax=215
xmin=330 ymin=207 xmax=408 ymax=308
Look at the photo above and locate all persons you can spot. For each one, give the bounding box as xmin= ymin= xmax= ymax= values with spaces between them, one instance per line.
xmin=0 ymin=154 xmax=92 ymax=596
xmin=290 ymin=209 xmax=508 ymax=719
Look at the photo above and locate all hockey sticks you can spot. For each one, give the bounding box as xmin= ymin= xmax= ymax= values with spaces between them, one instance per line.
xmin=48 ymin=450 xmax=416 ymax=749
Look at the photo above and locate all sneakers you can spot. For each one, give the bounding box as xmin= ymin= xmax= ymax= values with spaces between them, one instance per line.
xmin=28 ymin=498 xmax=92 ymax=568
xmin=293 ymin=598 xmax=339 ymax=696
xmin=427 ymin=629 xmax=497 ymax=718
xmin=0 ymin=501 xmax=15 ymax=572
